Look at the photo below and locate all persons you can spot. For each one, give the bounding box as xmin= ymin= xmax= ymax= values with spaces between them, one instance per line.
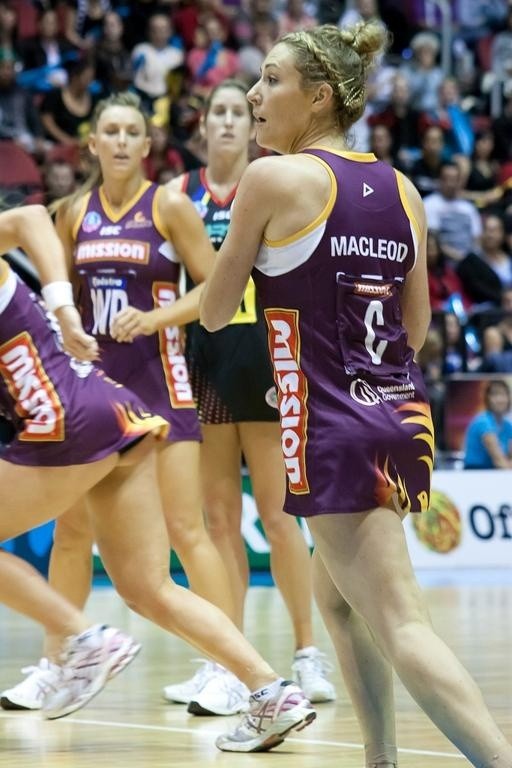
xmin=0 ymin=204 xmax=316 ymax=754
xmin=161 ymin=78 xmax=339 ymax=706
xmin=0 ymin=89 xmax=255 ymax=716
xmin=199 ymin=19 xmax=512 ymax=768
xmin=1 ymin=1 xmax=511 ymax=471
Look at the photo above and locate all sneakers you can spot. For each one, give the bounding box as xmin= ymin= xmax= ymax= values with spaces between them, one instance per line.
xmin=160 ymin=646 xmax=336 ymax=750
xmin=0 ymin=623 xmax=141 ymax=719
